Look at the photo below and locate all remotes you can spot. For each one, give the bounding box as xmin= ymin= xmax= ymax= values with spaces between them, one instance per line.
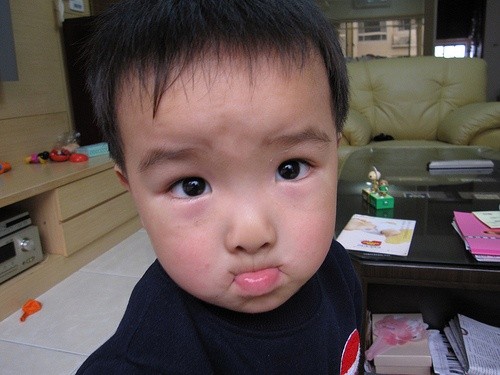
xmin=428 ymin=160 xmax=494 ymax=168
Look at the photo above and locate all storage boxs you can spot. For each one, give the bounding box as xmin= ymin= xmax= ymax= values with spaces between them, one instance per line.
xmin=371 ymin=314 xmax=432 ymax=375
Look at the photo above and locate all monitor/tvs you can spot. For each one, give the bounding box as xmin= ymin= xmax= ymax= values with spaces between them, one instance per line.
xmin=62 ymin=15 xmax=106 ymax=147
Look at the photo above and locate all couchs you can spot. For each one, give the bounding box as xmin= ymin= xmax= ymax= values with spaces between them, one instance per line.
xmin=337 ymin=57 xmax=500 ymax=189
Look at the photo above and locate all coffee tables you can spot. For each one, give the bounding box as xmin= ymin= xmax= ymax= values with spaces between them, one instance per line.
xmin=334 ymin=146 xmax=500 ymax=363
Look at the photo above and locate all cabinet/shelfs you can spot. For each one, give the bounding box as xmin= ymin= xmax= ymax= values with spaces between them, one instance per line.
xmin=63 ymin=16 xmax=108 ymax=148
xmin=0 ymin=148 xmax=142 ymax=322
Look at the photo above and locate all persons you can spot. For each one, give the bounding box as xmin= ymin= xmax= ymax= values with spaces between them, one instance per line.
xmin=75 ymin=0 xmax=364 ymax=375
